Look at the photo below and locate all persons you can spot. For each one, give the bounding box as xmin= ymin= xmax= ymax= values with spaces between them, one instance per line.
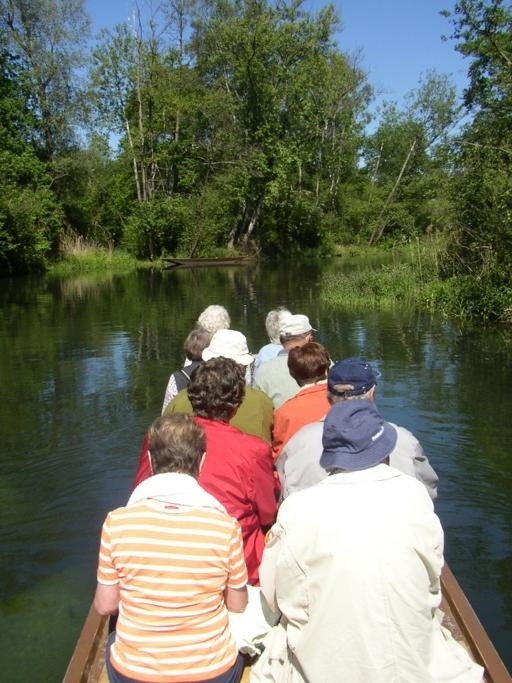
xmin=253 ymin=398 xmax=489 ymax=683
xmin=132 ymin=356 xmax=284 ymax=585
xmin=184 ymin=303 xmax=252 ymax=384
xmin=253 ymin=313 xmax=340 ymax=408
xmin=255 ymin=304 xmax=292 ymax=369
xmin=96 ymin=411 xmax=249 ymax=683
xmin=271 ymin=341 xmax=336 ymax=456
xmin=161 ymin=329 xmax=210 ymax=416
xmin=160 ymin=328 xmax=272 ymax=444
xmin=274 ymin=356 xmax=438 ymax=505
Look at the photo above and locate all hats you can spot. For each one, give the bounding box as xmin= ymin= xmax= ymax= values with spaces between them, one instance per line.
xmin=202 ymin=327 xmax=254 ymax=367
xmin=319 ymin=399 xmax=397 ymax=471
xmin=280 ymin=314 xmax=317 ymax=336
xmin=327 ymin=357 xmax=382 ymax=394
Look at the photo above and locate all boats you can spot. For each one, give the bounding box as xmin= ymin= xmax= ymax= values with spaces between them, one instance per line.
xmin=159 ymin=255 xmax=252 ymax=266
